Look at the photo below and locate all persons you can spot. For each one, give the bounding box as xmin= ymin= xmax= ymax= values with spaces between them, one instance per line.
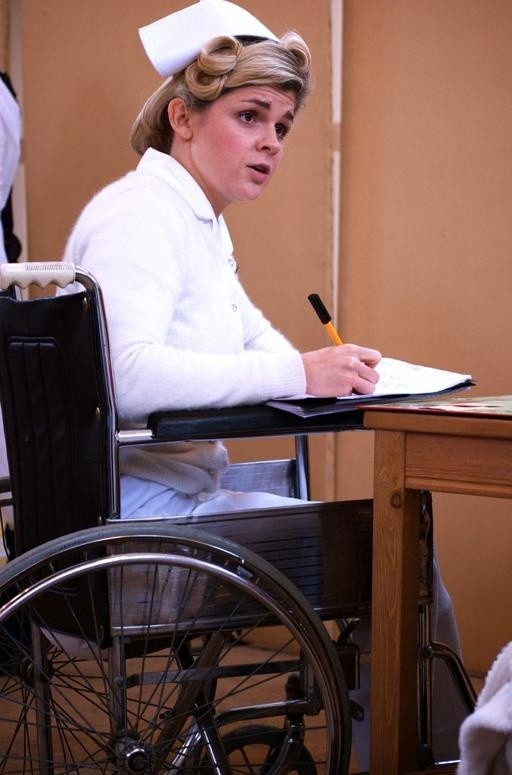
xmin=55 ymin=0 xmax=473 ymax=773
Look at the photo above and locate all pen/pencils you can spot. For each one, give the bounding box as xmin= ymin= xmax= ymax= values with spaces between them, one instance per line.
xmin=307 ymin=294 xmax=344 ymax=346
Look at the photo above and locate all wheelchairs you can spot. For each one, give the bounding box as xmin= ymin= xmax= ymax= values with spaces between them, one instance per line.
xmin=0 ymin=262 xmax=485 ymax=775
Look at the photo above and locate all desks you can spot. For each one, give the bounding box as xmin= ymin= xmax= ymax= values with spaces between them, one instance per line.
xmin=349 ymin=407 xmax=510 ymax=775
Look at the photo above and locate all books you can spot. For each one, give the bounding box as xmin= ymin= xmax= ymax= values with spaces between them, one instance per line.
xmin=265 ymin=354 xmax=473 ymax=419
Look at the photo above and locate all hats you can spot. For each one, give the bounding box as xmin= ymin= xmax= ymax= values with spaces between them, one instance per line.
xmin=137 ymin=1 xmax=281 ymax=78
